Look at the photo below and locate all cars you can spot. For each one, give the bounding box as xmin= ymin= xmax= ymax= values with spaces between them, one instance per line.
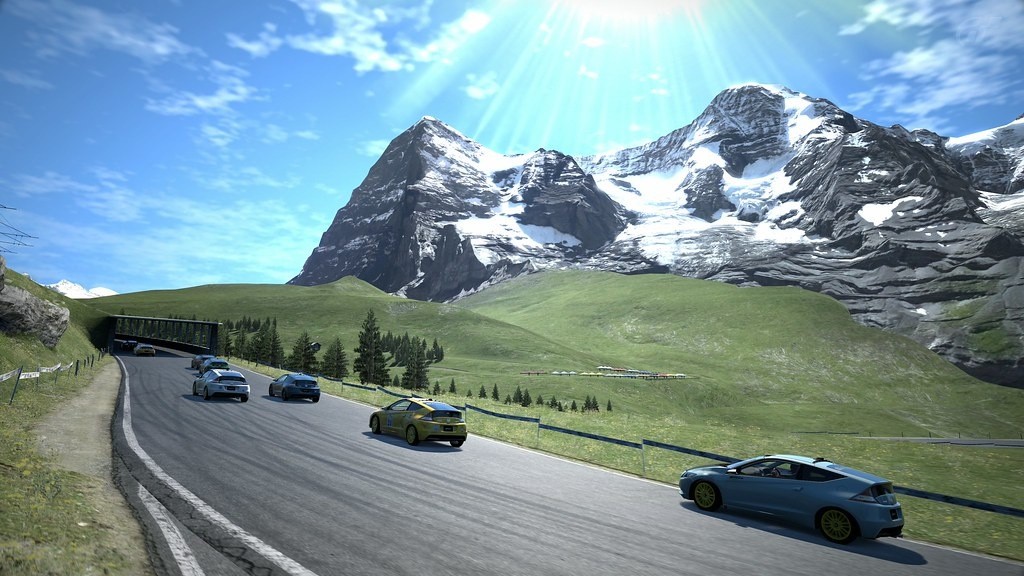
xmin=119 ymin=340 xmax=138 ymax=351
xmin=133 ymin=343 xmax=154 ymax=356
xmin=268 ymin=374 xmax=321 ymax=403
xmin=191 ymin=354 xmax=215 ymax=370
xmin=192 ymin=369 xmax=251 ymax=402
xmin=368 ymin=399 xmax=468 ymax=447
xmin=678 ymin=455 xmax=906 ymax=545
xmin=198 ymin=357 xmax=230 ymax=376
